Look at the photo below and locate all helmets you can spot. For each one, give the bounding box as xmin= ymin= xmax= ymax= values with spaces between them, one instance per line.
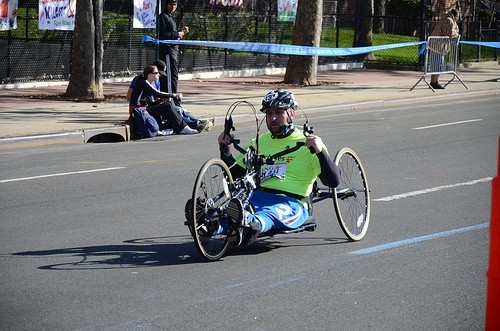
xmin=260 ymin=90 xmax=298 ymax=113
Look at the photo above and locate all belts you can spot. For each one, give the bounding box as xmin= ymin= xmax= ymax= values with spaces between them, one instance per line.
xmin=259 ymin=187 xmax=305 ymax=200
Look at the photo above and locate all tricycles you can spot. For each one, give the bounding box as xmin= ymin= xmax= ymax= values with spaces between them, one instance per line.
xmin=183 ymin=100 xmax=372 ymax=262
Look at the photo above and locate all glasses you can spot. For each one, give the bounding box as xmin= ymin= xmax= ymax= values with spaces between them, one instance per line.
xmin=151 ymin=72 xmax=158 ymax=74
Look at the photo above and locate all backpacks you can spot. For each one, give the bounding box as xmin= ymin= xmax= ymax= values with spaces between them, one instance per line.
xmin=134 ymin=107 xmax=159 ymax=137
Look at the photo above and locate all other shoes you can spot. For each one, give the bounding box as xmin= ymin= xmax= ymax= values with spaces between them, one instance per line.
xmin=198 ymin=120 xmax=207 ymax=133
xmin=179 ymin=125 xmax=199 ymax=134
xmin=429 ymin=82 xmax=445 ymax=89
xmin=184 ymin=198 xmax=204 ymax=239
xmin=228 ymin=198 xmax=248 ymax=246
xmin=206 ymin=118 xmax=215 ymax=131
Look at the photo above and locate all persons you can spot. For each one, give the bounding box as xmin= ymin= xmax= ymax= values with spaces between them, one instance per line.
xmin=428 ymin=8 xmax=459 ymax=89
xmin=129 ymin=65 xmax=197 ymax=137
xmin=126 ymin=60 xmax=215 ymax=133
xmin=185 ymin=91 xmax=341 ymax=249
xmin=156 ymin=0 xmax=189 ymax=104
